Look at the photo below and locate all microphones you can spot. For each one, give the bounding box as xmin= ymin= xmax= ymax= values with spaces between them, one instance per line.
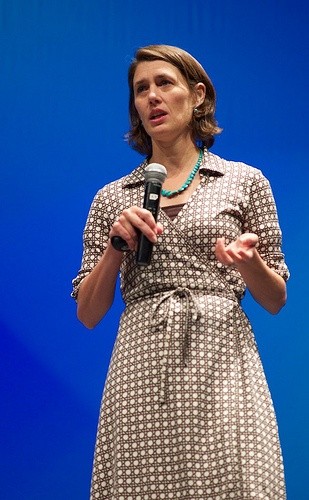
xmin=137 ymin=163 xmax=167 ymax=266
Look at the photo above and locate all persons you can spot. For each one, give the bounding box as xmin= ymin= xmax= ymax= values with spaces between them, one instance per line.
xmin=71 ymin=44 xmax=290 ymax=500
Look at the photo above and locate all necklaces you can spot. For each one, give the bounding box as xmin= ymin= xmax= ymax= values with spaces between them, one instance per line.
xmin=160 ymin=148 xmax=204 ymax=199
